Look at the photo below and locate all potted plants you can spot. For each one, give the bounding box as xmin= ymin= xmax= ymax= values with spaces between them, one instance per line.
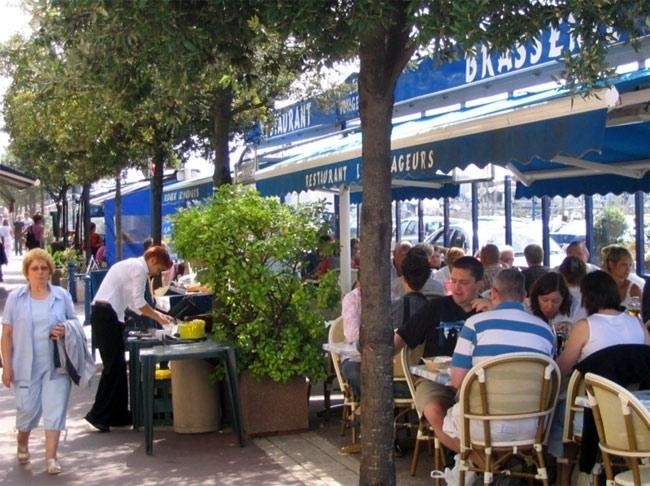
xmin=154 ymin=171 xmax=351 ymax=450
xmin=50 ymin=250 xmax=66 ymax=286
xmin=59 ymin=243 xmax=86 ymax=294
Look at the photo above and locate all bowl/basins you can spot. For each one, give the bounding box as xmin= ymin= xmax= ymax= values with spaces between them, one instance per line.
xmin=421 ymin=356 xmax=452 ymax=372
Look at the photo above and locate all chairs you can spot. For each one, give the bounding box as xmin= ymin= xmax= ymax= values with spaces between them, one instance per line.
xmin=316 ymin=264 xmax=650 ymax=486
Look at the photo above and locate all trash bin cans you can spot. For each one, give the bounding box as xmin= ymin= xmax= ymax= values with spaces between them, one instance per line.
xmin=170 ymin=357 xmax=223 ymax=434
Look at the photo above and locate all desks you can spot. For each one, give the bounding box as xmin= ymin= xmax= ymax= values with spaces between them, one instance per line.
xmin=133 ymin=336 xmax=250 ymax=459
xmin=118 ymin=326 xmax=221 ymax=431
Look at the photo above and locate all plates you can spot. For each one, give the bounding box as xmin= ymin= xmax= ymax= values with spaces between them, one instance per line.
xmin=129 ymin=331 xmax=151 ymax=338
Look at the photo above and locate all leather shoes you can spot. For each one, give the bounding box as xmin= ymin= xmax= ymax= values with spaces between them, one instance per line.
xmin=18 ymin=446 xmax=60 ymax=473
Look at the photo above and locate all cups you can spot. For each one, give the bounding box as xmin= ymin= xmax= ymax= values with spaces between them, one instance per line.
xmin=628 ymin=296 xmax=641 ymax=318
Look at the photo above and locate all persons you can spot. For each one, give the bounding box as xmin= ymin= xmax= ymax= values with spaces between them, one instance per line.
xmin=0 ymin=248 xmax=82 ymax=473
xmin=84 ymin=246 xmax=172 ymax=431
xmin=2 ymin=212 xmax=106 ymax=266
xmin=300 ymin=236 xmax=650 ymax=485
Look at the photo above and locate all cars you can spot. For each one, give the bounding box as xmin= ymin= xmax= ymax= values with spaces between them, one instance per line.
xmin=389 ymin=210 xmax=590 ymax=269
